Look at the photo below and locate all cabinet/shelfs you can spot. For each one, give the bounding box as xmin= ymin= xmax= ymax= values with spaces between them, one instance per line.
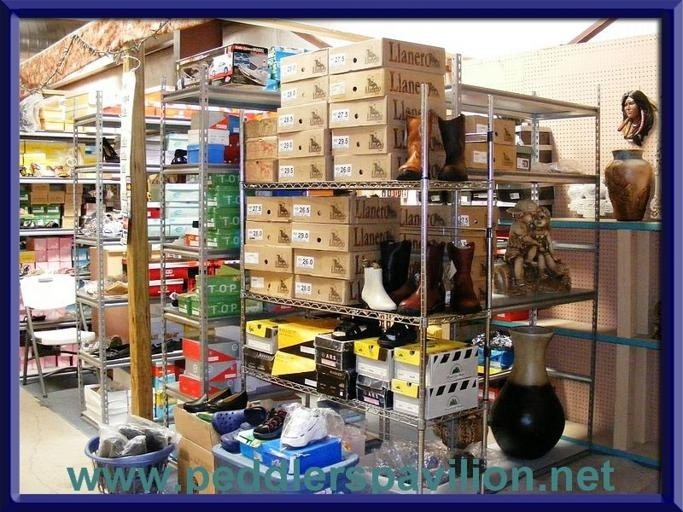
xmin=18 ymin=129 xmax=95 ymax=374
xmin=239 ymin=16 xmax=670 ymax=499
xmin=158 ymin=65 xmax=330 ymax=430
xmin=74 ymin=94 xmax=199 ymax=432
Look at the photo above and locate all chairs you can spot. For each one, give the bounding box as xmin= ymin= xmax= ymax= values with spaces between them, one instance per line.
xmin=20 ymin=273 xmax=96 ymax=398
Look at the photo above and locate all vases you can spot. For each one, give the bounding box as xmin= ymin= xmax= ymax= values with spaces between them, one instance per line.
xmin=490 ymin=325 xmax=566 ymax=461
xmin=605 ymin=149 xmax=655 ymax=221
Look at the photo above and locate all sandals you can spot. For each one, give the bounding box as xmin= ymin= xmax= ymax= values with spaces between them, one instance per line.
xmin=332 ymin=317 xmax=379 ymax=341
xmin=377 ymin=319 xmax=417 ymax=348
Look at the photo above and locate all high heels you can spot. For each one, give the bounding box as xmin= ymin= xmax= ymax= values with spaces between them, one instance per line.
xmin=102 ymin=137 xmax=121 ymax=162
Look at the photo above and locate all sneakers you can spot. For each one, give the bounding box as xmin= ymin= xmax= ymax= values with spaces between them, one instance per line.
xmin=253 ymin=410 xmax=289 ymax=440
xmin=280 ymin=411 xmax=328 ymax=449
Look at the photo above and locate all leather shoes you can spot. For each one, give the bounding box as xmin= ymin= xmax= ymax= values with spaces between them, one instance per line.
xmin=184 ymin=388 xmax=231 ymax=412
xmin=151 ymin=339 xmax=173 ymax=353
xmin=206 ymin=391 xmax=247 ymax=411
xmin=105 ymin=345 xmax=130 ymax=359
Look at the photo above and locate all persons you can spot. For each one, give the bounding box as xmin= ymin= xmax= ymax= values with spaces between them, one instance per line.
xmin=506 ymin=198 xmax=566 ymax=286
xmin=617 ymin=89 xmax=656 ymax=146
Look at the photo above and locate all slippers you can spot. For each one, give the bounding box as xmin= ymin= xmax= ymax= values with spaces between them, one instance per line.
xmin=212 ymin=407 xmax=268 ymax=434
xmin=220 ymin=422 xmax=259 ymax=454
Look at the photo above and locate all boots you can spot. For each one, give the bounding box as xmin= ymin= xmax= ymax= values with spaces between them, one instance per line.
xmin=449 ymin=242 xmax=482 ymax=313
xmin=397 ymin=243 xmax=445 ymax=315
xmin=397 ymin=116 xmax=431 ymax=180
xmin=363 ymin=242 xmax=410 ymax=309
xmin=361 ymin=267 xmax=396 ymax=313
xmin=437 ymin=114 xmax=468 ymax=181
xmin=389 ymin=263 xmax=421 ymax=303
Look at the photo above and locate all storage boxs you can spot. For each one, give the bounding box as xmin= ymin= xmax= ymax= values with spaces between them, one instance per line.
xmin=204 ymin=190 xmax=240 ymax=206
xmin=392 ymin=380 xmax=478 ymax=418
xmin=46 ymin=204 xmax=61 ymax=215
xmin=278 ymin=158 xmax=332 ymax=181
xmin=410 ymin=191 xmax=449 ymax=206
xmin=18 ymin=250 xmax=35 ymax=263
xmin=194 ymin=275 xmax=240 ymax=295
xmin=35 ymin=250 xmax=48 ymax=262
xmin=400 ymin=225 xmax=497 ymax=256
xmin=270 ymin=350 xmax=318 ymax=389
xmin=293 ymin=249 xmax=384 ymax=280
xmin=280 ymin=49 xmax=329 ymax=82
xmin=48 ymin=251 xmax=60 ymax=262
xmin=330 ymin=125 xmax=407 ymax=155
xmin=163 ymin=134 xmax=187 ymax=151
xmin=20 ymin=191 xmax=30 ymax=203
xmin=178 ymin=293 xmax=198 ymax=314
xmin=175 ymin=44 xmax=270 ymax=88
xmin=206 ymin=174 xmax=240 ymax=189
xmin=352 ymin=446 xmax=479 ymax=493
xmin=62 ymin=205 xmax=82 ymax=217
xmin=148 ymin=279 xmax=184 ymax=295
xmin=330 ymin=37 xmax=446 ymax=73
xmin=393 ymin=339 xmax=479 ymax=385
xmin=30 ymin=190 xmax=48 ymax=204
xmin=163 ymin=202 xmax=200 ymax=217
xmin=82 ymin=203 xmax=96 ymax=214
xmin=73 ymin=262 xmax=90 ymax=272
xmin=281 ymin=75 xmax=330 ymax=106
xmin=268 ymin=45 xmax=294 ymax=81
xmin=190 ymin=112 xmax=229 ymax=130
xmin=40 ymin=118 xmax=65 ymax=131
xmin=354 ymin=374 xmax=392 ymax=408
xmin=92 ymin=303 xmax=130 ymax=346
xmin=243 ymin=345 xmax=275 ymax=375
xmin=249 ymin=270 xmax=294 ymax=299
xmin=228 ymin=116 xmax=240 ymax=131
xmin=178 ymin=373 xmax=238 ymax=399
xmin=164 ymin=217 xmax=200 ymax=238
xmin=148 ymin=263 xmax=188 ymax=279
xmin=89 ymin=247 xmax=124 ymax=278
xmin=64 ymin=108 xmax=88 ymax=121
xmin=20 ymin=217 xmax=33 ymax=228
xmin=294 ymin=275 xmax=365 ymax=305
xmin=278 ymin=128 xmax=331 ymax=157
xmin=330 ymin=66 xmax=445 ymax=99
xmin=33 ymin=216 xmax=45 ymax=227
xmin=212 ymin=441 xmax=359 ymax=496
xmin=191 ymin=296 xmax=239 ymax=316
xmin=72 ymin=248 xmax=89 ymax=262
xmin=515 ymin=125 xmax=550 ymax=144
xmin=31 ymin=204 xmax=46 ymax=215
xmin=491 ymin=308 xmax=530 ymax=319
xmin=244 ymin=136 xmax=277 ymax=160
xmin=83 ymin=379 xmax=129 ymax=423
xmin=65 ymin=93 xmax=91 ymax=109
xmin=236 ymin=427 xmax=343 ymax=473
xmin=187 ymin=130 xmax=229 ymax=147
xmin=203 ymin=206 xmax=239 ymax=229
xmin=65 ymin=121 xmax=83 ymax=132
xmin=401 ymin=204 xmax=497 ymax=229
xmin=277 ymin=319 xmax=347 ymax=359
xmin=242 ymin=117 xmax=278 ymax=137
xmin=39 ymin=106 xmax=63 ymax=118
xmin=352 ymin=333 xmax=424 ymax=380
xmin=463 ymin=115 xmax=516 ymax=143
xmin=20 ymin=204 xmax=30 ymax=216
xmin=292 ymin=195 xmax=401 ymax=225
xmin=181 ymin=333 xmax=240 ymax=361
xmin=204 ymin=229 xmax=239 ymax=248
xmin=60 ymin=238 xmax=71 ymax=250
xmin=244 ymin=222 xmax=292 ymax=246
xmin=184 ymin=358 xmax=242 ymax=382
xmin=18 ymin=139 xmax=92 ymax=176
xmin=19 ymin=264 xmax=35 ymax=274
xmin=315 ymin=365 xmax=356 ymax=400
xmin=47 ymin=237 xmax=59 ymax=250
xmin=146 ymin=201 xmax=162 ymax=218
xmin=277 ymin=101 xmax=328 ymax=133
xmin=242 ymin=243 xmax=294 ymax=274
xmin=459 ymin=191 xmax=472 ymax=206
xmin=498 ymin=186 xmax=555 ymax=207
xmin=65 ymin=194 xmax=82 ymax=204
xmin=148 ymin=240 xmax=170 ymax=250
xmin=473 ymin=189 xmax=496 ymax=206
xmin=291 ymin=222 xmax=400 ymax=252
xmin=314 ymin=331 xmax=356 ymax=372
xmin=516 ymin=144 xmax=554 ymax=173
xmin=35 ymin=262 xmax=49 ymax=273
xmin=465 ymin=142 xmax=516 ymax=172
xmin=187 ymin=144 xmax=224 ymax=164
xmin=147 ymin=218 xmax=164 ymax=238
xmin=60 ymin=250 xmax=72 ymax=261
xmin=334 ymin=155 xmax=407 ymax=180
xmin=50 ymin=263 xmax=60 ymax=274
xmin=48 ymin=191 xmax=64 ymax=204
xmin=61 ymin=217 xmax=76 ymax=229
xmin=26 ymin=183 xmax=49 ymax=191
xmin=164 ymin=184 xmax=200 ymax=203
xmin=173 ymin=402 xmax=225 ymax=496
xmin=26 ymin=237 xmax=47 ymax=250
xmin=245 ymin=316 xmax=310 ymax=355
xmin=60 ymin=261 xmax=72 ymax=274
xmin=65 ymin=183 xmax=82 ymax=193
xmin=243 ymin=158 xmax=278 ymax=182
xmin=46 ymin=217 xmax=61 ymax=228
xmin=164 ymin=150 xmax=186 ymax=164
xmin=246 ymin=196 xmax=292 ymax=221
xmin=329 ymin=95 xmax=447 ymax=128
